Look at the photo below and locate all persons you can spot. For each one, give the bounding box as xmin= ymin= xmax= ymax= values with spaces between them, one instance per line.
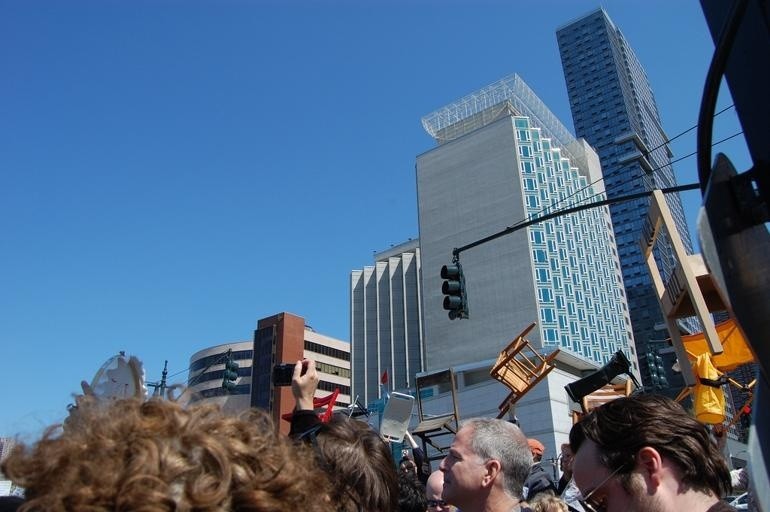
xmin=0 ymin=354 xmax=770 ymax=512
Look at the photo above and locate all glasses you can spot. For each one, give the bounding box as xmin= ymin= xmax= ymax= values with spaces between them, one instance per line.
xmin=577 ymin=457 xmax=632 ymax=512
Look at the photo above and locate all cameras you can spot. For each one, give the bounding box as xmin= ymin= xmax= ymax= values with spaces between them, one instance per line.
xmin=272 ymin=364 xmax=307 ymax=386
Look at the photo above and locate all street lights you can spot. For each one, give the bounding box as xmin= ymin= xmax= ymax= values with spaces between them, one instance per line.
xmin=222 ymin=361 xmax=239 ymax=393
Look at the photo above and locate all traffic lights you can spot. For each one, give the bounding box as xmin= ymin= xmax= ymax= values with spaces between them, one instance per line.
xmin=439 ymin=264 xmax=469 ymax=320
